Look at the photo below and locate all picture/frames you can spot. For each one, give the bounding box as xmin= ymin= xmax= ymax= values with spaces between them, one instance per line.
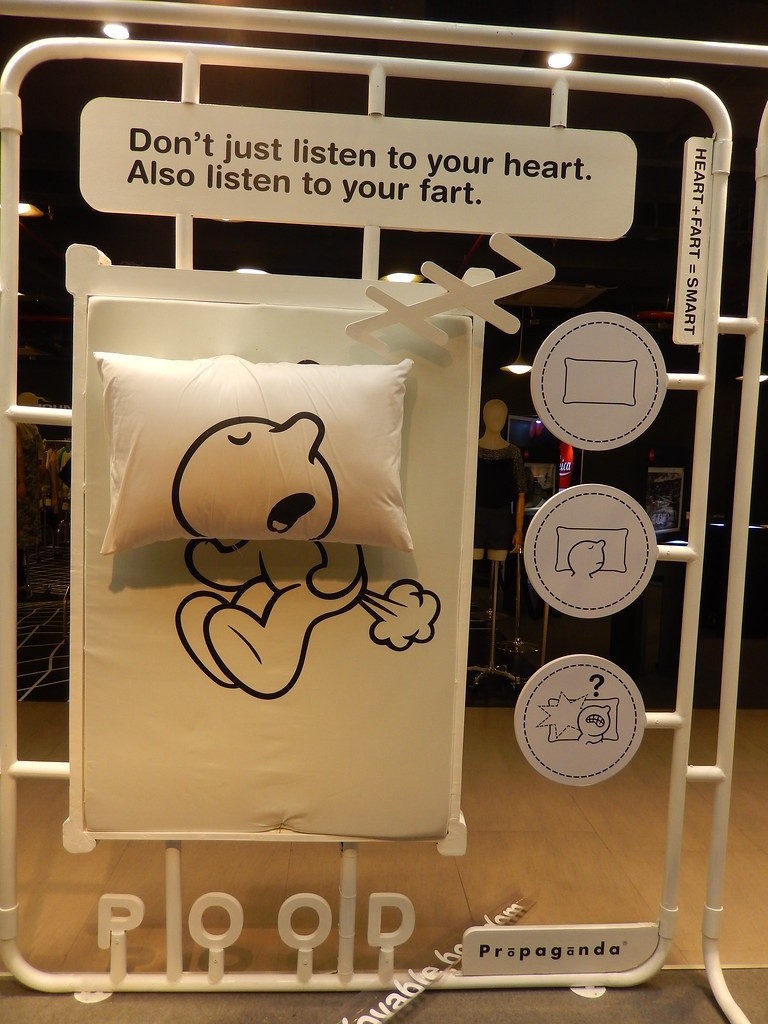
xmin=507 ymin=415 xmax=558 ymax=515
xmin=646 ymin=468 xmax=685 ymax=533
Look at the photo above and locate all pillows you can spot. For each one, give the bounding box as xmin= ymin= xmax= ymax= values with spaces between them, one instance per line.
xmin=92 ymin=353 xmax=418 ymax=548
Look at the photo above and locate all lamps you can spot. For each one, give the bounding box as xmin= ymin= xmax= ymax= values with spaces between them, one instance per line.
xmin=379 ymin=263 xmax=425 ymax=283
xmin=499 ymin=308 xmax=533 ymax=375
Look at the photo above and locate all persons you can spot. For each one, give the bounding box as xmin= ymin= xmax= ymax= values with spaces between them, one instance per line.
xmin=473 ymin=400 xmax=526 ymax=562
xmin=16 ymin=392 xmax=47 ymax=554
